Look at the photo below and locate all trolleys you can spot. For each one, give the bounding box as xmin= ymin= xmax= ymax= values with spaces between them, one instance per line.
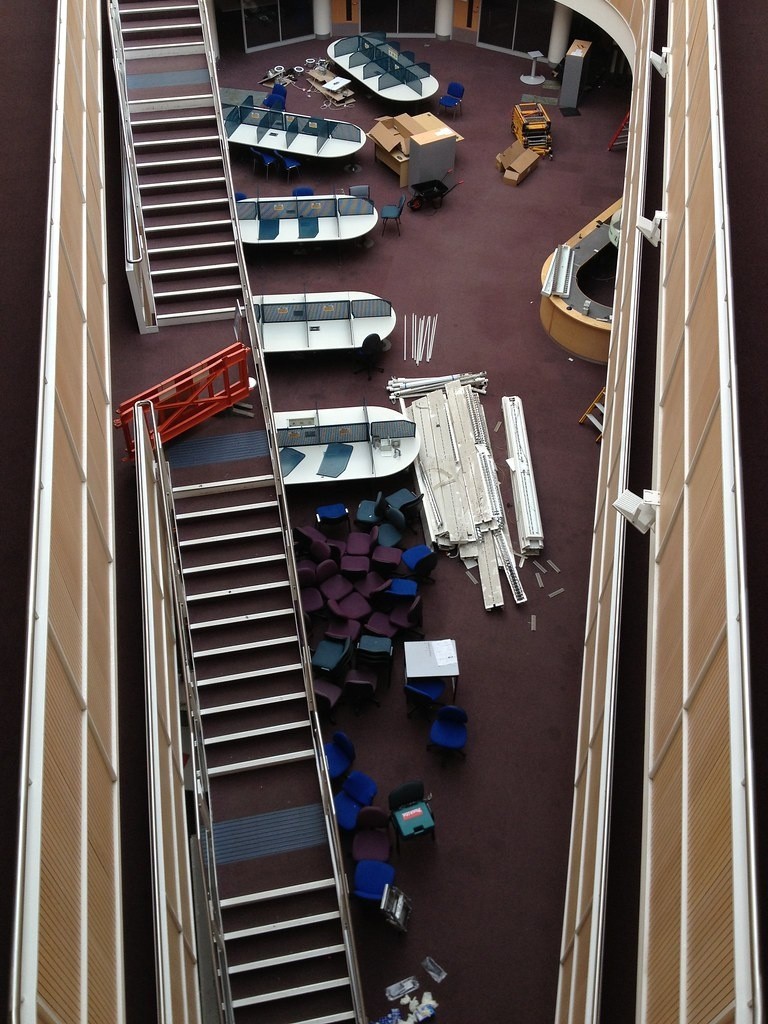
xmin=406 ymin=169 xmax=465 ymax=212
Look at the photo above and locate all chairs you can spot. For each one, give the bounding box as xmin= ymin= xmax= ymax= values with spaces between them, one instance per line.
xmin=274 ymin=150 xmax=300 ymax=184
xmin=349 ymin=184 xmax=370 ymax=199
xmin=292 ymin=186 xmax=314 ymax=196
xmin=380 ymin=192 xmax=406 ymax=237
xmin=250 ymin=147 xmax=274 ymax=180
xmin=426 ymin=705 xmax=469 ymax=762
xmin=263 ymin=84 xmax=287 ymax=111
xmin=334 ymin=769 xmax=377 ymax=830
xmin=324 ymin=732 xmax=356 ymax=778
xmin=350 ymin=807 xmax=393 ymax=862
xmin=353 ymin=860 xmax=394 ymax=900
xmin=405 ymin=671 xmax=446 ymax=715
xmin=384 ymin=578 xmax=417 ymax=597
xmin=389 ymin=596 xmax=426 ymax=638
xmin=437 ymin=82 xmax=465 ymax=122
xmin=387 ymin=781 xmax=437 ymax=855
xmin=235 ymin=192 xmax=248 ymax=202
xmin=384 ymin=488 xmax=424 ymax=517
xmin=291 ymin=491 xmax=401 ymax=723
xmin=401 ymin=545 xmax=438 ymax=588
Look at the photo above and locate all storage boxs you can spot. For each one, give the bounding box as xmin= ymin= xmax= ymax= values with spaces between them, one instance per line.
xmin=369 ymin=113 xmax=428 ymax=155
xmin=500 ymin=140 xmax=540 ymax=187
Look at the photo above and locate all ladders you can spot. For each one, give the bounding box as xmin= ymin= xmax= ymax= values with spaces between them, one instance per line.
xmin=606 ymin=110 xmax=630 ymax=152
xmin=510 ymin=102 xmax=554 ymax=160
xmin=579 ymin=386 xmax=607 ymax=443
xmin=114 ymin=340 xmax=256 ymax=464
xmin=380 ymin=883 xmax=412 ymax=932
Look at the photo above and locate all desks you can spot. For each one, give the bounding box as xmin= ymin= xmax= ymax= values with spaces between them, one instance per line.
xmin=236 ymin=185 xmax=378 ymax=244
xmin=326 ymin=31 xmax=440 ymax=101
xmin=222 ymin=95 xmax=366 ymax=158
xmin=252 ymin=291 xmax=397 ymax=353
xmin=520 ymin=51 xmax=546 ymax=85
xmin=273 ymin=397 xmax=422 ymax=485
xmin=366 ymin=112 xmax=464 ymax=188
